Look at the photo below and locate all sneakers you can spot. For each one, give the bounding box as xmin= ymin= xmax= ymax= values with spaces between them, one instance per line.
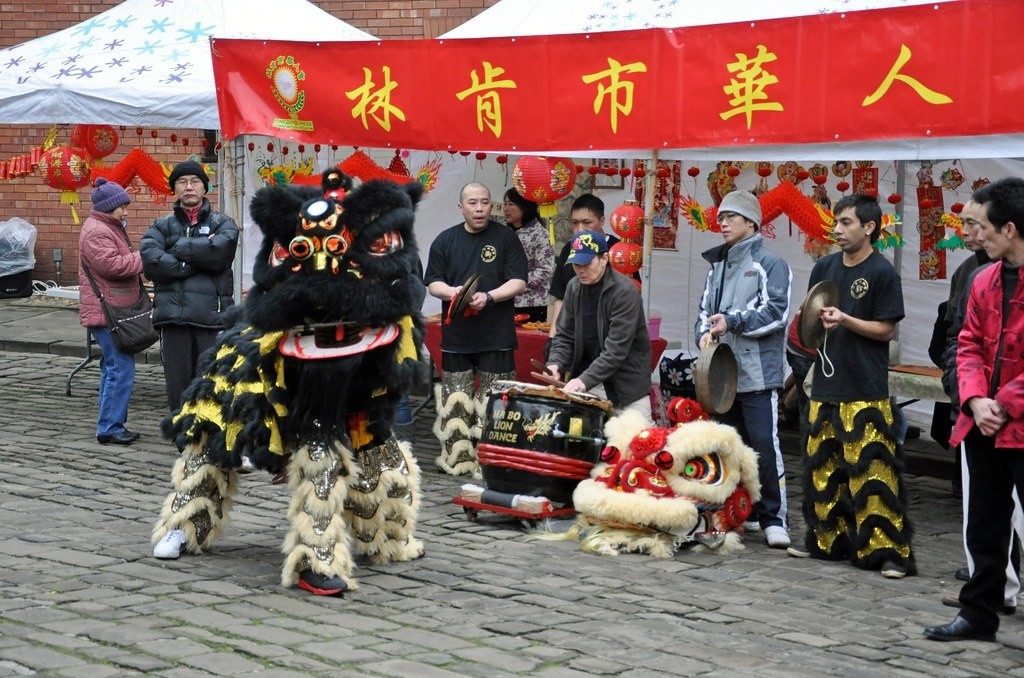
xmin=298 ymin=569 xmax=348 ymax=595
xmin=154 ymin=530 xmax=186 ymax=558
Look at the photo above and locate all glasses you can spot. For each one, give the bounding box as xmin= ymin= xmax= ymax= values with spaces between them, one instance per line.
xmin=714 ymin=211 xmax=737 ymax=225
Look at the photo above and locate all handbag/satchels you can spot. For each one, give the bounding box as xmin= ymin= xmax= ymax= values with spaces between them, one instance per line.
xmin=107 ymin=291 xmax=160 ymax=354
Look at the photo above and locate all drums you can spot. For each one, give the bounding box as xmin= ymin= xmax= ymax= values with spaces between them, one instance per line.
xmin=478 ymin=379 xmax=606 ymax=504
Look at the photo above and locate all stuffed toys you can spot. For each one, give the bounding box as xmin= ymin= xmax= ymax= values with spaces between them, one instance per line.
xmin=570 ymin=398 xmax=761 ymax=557
xmin=152 ymin=170 xmax=424 ymax=595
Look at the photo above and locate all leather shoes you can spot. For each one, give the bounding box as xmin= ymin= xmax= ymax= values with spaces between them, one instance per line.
xmin=923 ymin=616 xmax=997 ymax=643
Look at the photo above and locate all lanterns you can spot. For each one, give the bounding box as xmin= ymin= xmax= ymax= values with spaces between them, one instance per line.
xmin=71 ymin=124 xmax=118 ymax=168
xmin=247 ymin=140 xmax=359 ymax=156
xmin=951 ymin=203 xmax=964 ymax=213
xmin=609 ymin=200 xmax=645 ymax=242
xmin=119 ymin=125 xmax=222 ymax=154
xmin=814 ymin=175 xmax=877 ymax=197
xmin=609 ymin=241 xmax=643 ymax=278
xmin=448 ymin=150 xmax=508 ymax=170
xmin=512 ymin=155 xmax=575 ymax=244
xmin=888 ymin=193 xmax=901 ymax=204
xmin=920 ymin=199 xmax=933 ymax=209
xmin=727 ymin=167 xmax=809 ymax=180
xmin=241 ymin=0 xmax=1024 ymax=375
xmin=575 ymin=164 xmax=699 ymax=178
xmin=38 ymin=142 xmax=90 ymax=205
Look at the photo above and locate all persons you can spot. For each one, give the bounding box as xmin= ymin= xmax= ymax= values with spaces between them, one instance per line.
xmin=422 ymin=181 xmax=528 ymax=477
xmin=787 ymin=194 xmax=918 ymax=578
xmin=544 ymin=194 xmax=641 ymax=363
xmin=543 ymin=230 xmax=653 ymax=425
xmin=78 ymin=178 xmax=145 ymax=444
xmin=923 ymin=176 xmax=1024 ymax=641
xmin=504 ymin=187 xmax=554 ymax=322
xmin=139 ymin=161 xmax=239 ymax=412
xmin=694 ymin=190 xmax=792 ymax=548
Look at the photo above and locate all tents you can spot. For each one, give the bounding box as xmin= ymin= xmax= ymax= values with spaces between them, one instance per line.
xmin=0 ymin=0 xmax=382 ymax=214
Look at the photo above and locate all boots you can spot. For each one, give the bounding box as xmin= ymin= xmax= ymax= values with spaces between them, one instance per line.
xmin=394 ymin=418 xmax=417 ymax=447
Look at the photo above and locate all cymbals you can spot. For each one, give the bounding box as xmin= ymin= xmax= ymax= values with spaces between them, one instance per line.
xmin=449 ymin=271 xmax=486 ymax=321
xmin=796 ymin=280 xmax=841 ymax=350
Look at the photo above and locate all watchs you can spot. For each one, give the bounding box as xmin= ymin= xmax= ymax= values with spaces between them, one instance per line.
xmin=485 ymin=290 xmax=494 ymax=309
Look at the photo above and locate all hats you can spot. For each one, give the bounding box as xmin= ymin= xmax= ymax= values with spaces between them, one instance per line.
xmin=91 ymin=177 xmax=131 ymax=213
xmin=564 ymin=229 xmax=609 ymax=265
xmin=168 ymin=160 xmax=209 ymax=195
xmin=718 ymin=189 xmax=762 ymax=230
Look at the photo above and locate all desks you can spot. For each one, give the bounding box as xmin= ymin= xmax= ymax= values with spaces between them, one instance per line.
xmin=888 ymin=364 xmax=951 ymax=414
xmin=43 ymin=286 xmax=156 ymax=397
xmin=411 ymin=313 xmax=668 ymax=417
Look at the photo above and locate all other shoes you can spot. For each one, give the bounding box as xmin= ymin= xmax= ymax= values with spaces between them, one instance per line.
xmin=779 ymin=400 xmax=800 ymax=425
xmin=955 ymin=567 xmax=972 ymax=582
xmin=786 ymin=545 xmax=813 ymax=558
xmin=416 ymin=549 xmax=425 ymax=558
xmin=942 ymin=593 xmax=1016 ymax=616
xmin=272 ymin=471 xmax=290 ymax=484
xmin=235 ymin=455 xmax=254 ymax=472
xmin=741 ymin=521 xmax=761 ymax=533
xmin=765 ymin=524 xmax=790 ymax=546
xmin=777 ymin=384 xmax=789 ymax=399
xmin=880 ymin=560 xmax=907 ymax=577
xmin=97 ymin=429 xmax=141 ymax=444
